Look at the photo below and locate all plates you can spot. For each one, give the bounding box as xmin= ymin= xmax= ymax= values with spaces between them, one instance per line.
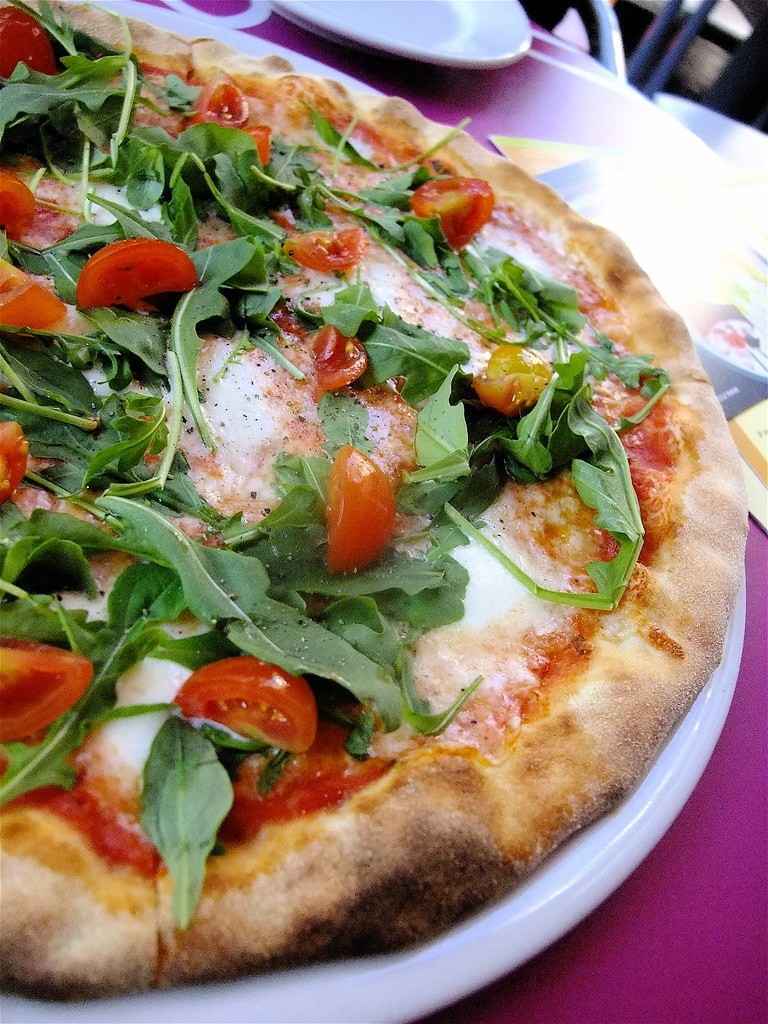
xmin=0 ymin=1 xmax=747 ymax=1024
xmin=267 ymin=0 xmax=532 ymax=67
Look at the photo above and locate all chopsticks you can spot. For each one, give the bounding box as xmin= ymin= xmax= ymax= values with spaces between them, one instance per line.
xmin=628 ymin=0 xmax=715 ymax=98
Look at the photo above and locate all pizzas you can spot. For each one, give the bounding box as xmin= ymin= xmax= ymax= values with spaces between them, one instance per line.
xmin=0 ymin=0 xmax=745 ymax=1000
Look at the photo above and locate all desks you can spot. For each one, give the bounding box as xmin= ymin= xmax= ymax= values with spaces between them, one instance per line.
xmin=96 ymin=0 xmax=768 ymax=1023
